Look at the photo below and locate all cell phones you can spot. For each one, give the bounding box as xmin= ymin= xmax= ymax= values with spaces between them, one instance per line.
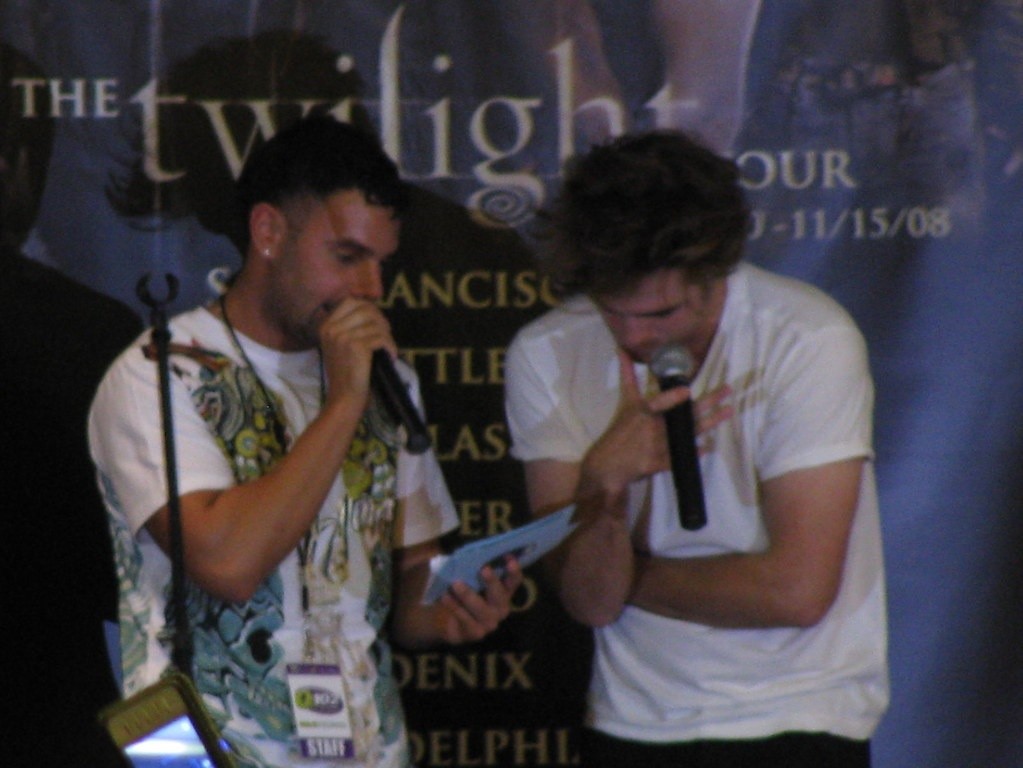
xmin=100 ymin=671 xmax=238 ymax=768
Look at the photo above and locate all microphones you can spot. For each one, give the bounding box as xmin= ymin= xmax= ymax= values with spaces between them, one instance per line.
xmin=367 ymin=349 xmax=431 ymax=457
xmin=651 ymin=344 xmax=708 ymax=531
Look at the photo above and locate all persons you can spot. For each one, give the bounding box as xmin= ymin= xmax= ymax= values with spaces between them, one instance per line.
xmin=506 ymin=132 xmax=892 ymax=768
xmin=87 ymin=114 xmax=522 ymax=767
